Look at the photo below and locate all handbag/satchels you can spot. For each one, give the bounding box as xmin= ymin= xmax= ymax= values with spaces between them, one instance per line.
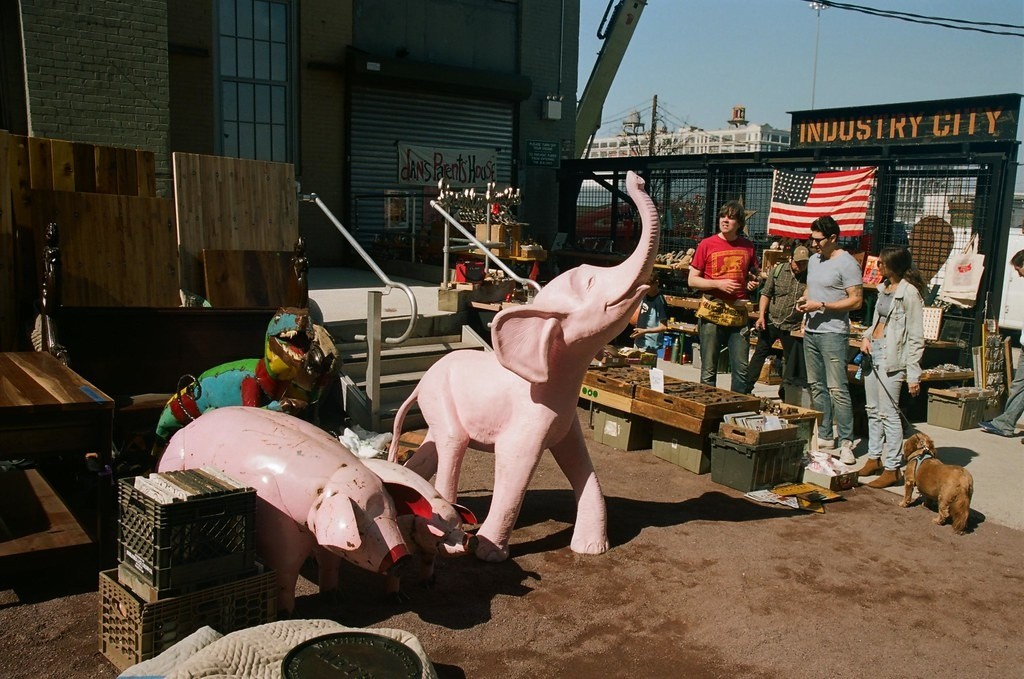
xmin=938 ymin=234 xmax=985 ymax=308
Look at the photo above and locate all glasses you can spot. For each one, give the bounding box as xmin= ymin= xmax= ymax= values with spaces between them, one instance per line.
xmin=809 ymin=233 xmax=829 ymax=242
xmin=877 ymin=260 xmax=882 ymax=268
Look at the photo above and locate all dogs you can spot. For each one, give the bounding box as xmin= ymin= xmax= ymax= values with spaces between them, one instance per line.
xmin=898 ymin=433 xmax=974 ymax=536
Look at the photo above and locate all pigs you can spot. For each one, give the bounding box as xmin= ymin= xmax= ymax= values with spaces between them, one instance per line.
xmin=156 ymin=406 xmax=479 ymax=620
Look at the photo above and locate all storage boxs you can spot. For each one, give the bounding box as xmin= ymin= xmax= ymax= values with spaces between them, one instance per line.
xmin=477 ymin=224 xmax=546 ymax=258
xmin=97 ymin=468 xmax=276 ymax=673
xmin=582 ymin=293 xmax=997 ymax=494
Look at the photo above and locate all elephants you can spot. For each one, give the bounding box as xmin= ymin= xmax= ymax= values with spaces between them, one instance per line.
xmin=383 ymin=170 xmax=667 ymax=560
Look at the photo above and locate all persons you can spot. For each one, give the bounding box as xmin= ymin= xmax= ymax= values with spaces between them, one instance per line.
xmin=746 ymin=245 xmax=809 ymax=403
xmin=630 ymin=272 xmax=669 ymax=353
xmin=688 ymin=201 xmax=763 ymax=395
xmin=795 ymin=216 xmax=863 ymax=463
xmin=977 ymin=249 xmax=1024 ymax=437
xmin=858 ymin=247 xmax=925 ymax=487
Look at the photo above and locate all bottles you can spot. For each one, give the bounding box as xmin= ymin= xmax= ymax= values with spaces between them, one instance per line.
xmin=682 ymin=352 xmax=685 ymax=363
xmin=511 ymin=289 xmax=517 ymax=302
xmin=664 ymin=345 xmax=670 ymax=361
xmin=670 ymin=338 xmax=679 ymax=363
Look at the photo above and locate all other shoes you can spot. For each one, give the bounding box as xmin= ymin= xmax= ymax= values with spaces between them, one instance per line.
xmin=868 ymin=467 xmax=905 ymax=488
xmin=978 ymin=420 xmax=1014 ymax=437
xmin=857 ymin=458 xmax=884 ymax=476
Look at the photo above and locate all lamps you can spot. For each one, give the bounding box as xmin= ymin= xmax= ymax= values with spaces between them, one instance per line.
xmin=541 ymin=94 xmax=567 ymax=120
xmin=396 ymin=45 xmax=409 ymax=56
xmin=344 ymin=44 xmax=367 ymax=62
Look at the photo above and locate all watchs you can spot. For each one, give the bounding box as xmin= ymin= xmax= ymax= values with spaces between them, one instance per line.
xmin=820 ymin=302 xmax=825 ymax=311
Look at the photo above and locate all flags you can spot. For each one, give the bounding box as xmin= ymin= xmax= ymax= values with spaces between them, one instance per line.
xmin=769 ymin=167 xmax=877 ymax=239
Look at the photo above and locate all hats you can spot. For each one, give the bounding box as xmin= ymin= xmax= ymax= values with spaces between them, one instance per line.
xmin=793 ymin=245 xmax=810 ymax=262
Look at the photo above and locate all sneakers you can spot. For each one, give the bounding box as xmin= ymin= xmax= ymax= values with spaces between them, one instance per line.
xmin=810 ymin=436 xmax=836 ymax=448
xmin=840 ymin=440 xmax=856 ymax=465
